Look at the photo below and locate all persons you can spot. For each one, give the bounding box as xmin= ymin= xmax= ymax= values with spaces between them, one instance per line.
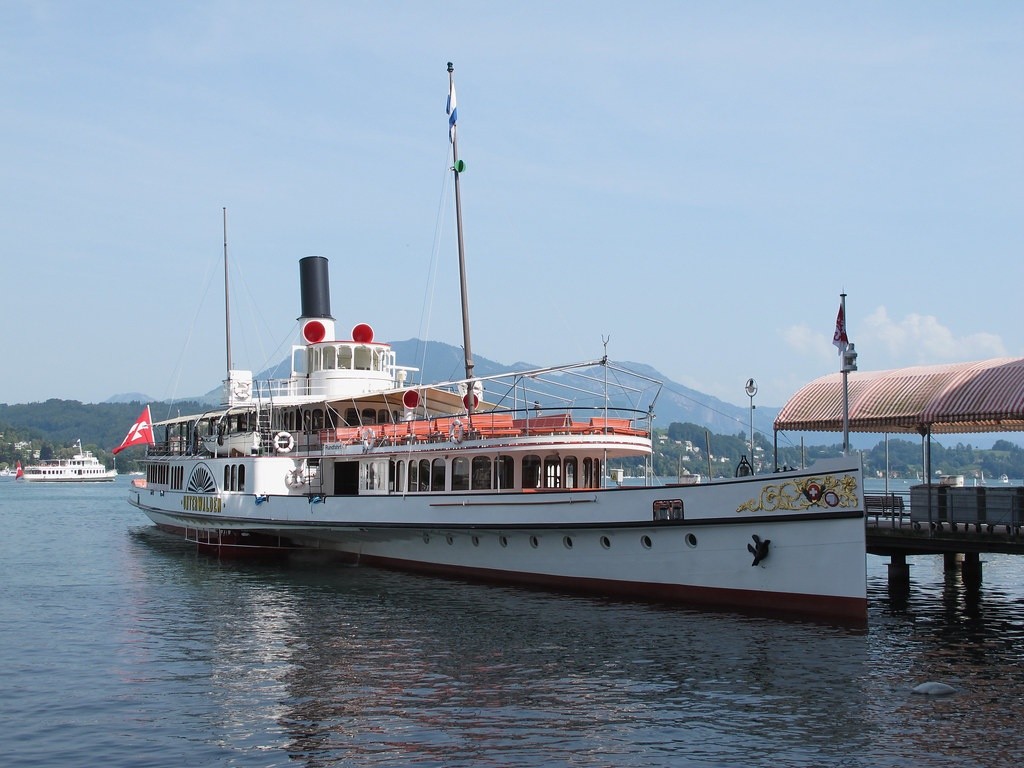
xmin=473 ymin=463 xmax=491 ymax=488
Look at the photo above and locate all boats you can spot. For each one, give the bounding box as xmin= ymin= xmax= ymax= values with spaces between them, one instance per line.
xmin=997 ymin=474 xmax=1009 ymax=484
xmin=0 ymin=466 xmax=18 ymax=476
xmin=902 ymin=480 xmax=907 ymax=484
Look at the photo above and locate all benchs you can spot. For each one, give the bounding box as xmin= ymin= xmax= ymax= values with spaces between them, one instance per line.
xmin=864 ymin=495 xmax=911 ymax=528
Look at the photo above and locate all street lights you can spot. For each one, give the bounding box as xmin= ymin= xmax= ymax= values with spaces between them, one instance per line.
xmin=744 ymin=377 xmax=758 ymax=480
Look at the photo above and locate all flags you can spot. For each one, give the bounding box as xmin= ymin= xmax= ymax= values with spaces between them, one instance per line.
xmin=446 ymin=76 xmax=458 ymax=143
xmin=16 ymin=460 xmax=23 ymax=480
xmin=111 ymin=406 xmax=154 ymax=455
xmin=832 ymin=303 xmax=849 ymax=356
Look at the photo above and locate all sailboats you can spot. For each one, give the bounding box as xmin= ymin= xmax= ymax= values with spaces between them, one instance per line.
xmin=915 ymin=470 xmax=923 ymax=482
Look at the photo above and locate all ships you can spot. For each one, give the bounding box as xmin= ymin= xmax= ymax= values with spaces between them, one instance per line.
xmin=15 ymin=439 xmax=119 ymax=482
xmin=112 ymin=61 xmax=872 ymax=633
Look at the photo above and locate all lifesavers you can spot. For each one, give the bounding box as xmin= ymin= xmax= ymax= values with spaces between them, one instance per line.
xmin=363 ymin=429 xmax=376 ymax=449
xmin=286 ymin=467 xmax=307 ymax=488
xmin=450 ymin=420 xmax=463 ymax=444
xmin=274 ymin=431 xmax=295 ymax=454
